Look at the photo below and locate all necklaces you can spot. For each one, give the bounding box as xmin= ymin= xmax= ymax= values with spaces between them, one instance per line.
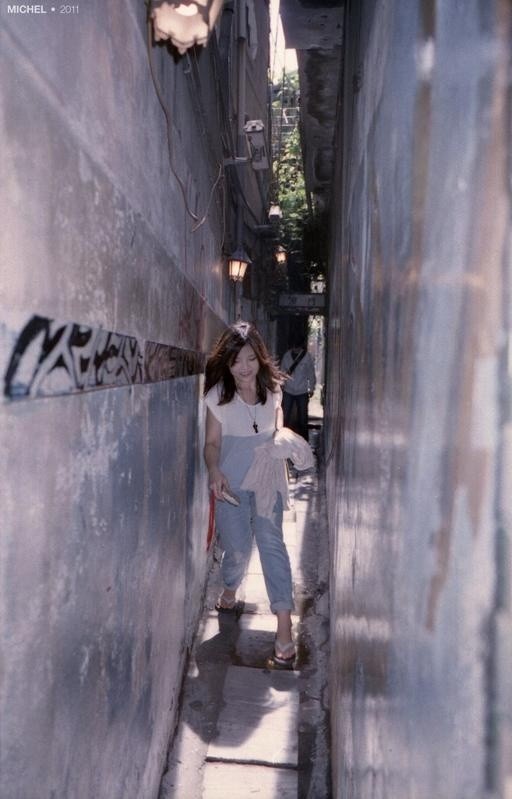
xmin=240 ymin=389 xmax=258 ymax=434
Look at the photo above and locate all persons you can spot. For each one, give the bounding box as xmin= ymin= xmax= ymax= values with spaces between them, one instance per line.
xmin=202 ymin=321 xmax=315 ymax=666
xmin=281 ymin=337 xmax=317 ymax=442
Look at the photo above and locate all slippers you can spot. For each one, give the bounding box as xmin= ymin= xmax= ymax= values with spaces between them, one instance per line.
xmin=271 ymin=631 xmax=297 ymax=666
xmin=214 ymin=592 xmax=237 ymax=613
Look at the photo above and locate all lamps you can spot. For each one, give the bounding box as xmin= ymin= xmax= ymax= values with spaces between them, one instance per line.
xmin=143 ymin=1 xmax=229 ymax=61
xmin=223 ymin=240 xmax=253 ymax=285
xmin=273 ymin=243 xmax=287 ymax=266
